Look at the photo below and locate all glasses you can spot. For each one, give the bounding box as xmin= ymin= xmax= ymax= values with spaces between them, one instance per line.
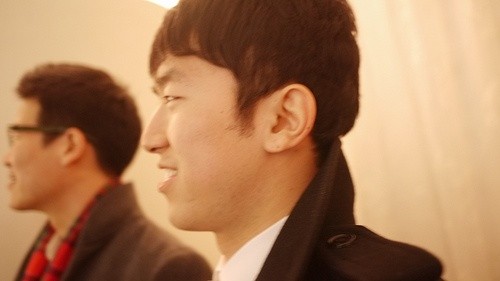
xmin=7 ymin=126 xmax=100 ymax=148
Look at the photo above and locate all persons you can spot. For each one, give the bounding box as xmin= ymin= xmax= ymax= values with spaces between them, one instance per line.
xmin=4 ymin=63 xmax=213 ymax=281
xmin=142 ymin=0 xmax=445 ymax=281
